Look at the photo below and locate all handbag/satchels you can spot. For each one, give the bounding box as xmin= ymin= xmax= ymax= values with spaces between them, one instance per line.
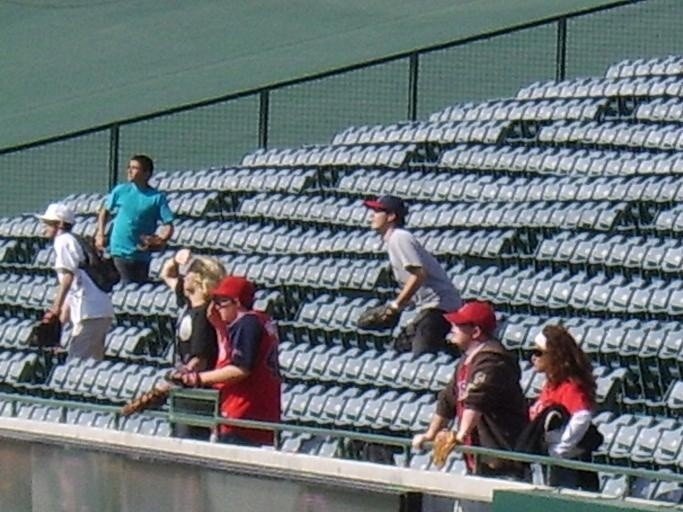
xmin=545 ymin=411 xmax=588 ymax=460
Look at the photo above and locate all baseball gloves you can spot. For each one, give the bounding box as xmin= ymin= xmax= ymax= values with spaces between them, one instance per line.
xmin=164 ymin=366 xmax=201 ymax=388
xmin=137 ymin=235 xmax=168 ymax=253
xmin=433 ymin=428 xmax=457 ymax=470
xmin=28 ymin=313 xmax=61 ymax=347
xmin=358 ymin=300 xmax=403 ymax=332
xmin=122 ymin=388 xmax=168 ymax=418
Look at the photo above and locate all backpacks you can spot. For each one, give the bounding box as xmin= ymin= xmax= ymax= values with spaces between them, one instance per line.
xmin=60 ymin=231 xmax=121 ymax=293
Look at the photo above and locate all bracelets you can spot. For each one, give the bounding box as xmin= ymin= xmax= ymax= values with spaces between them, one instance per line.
xmin=172 ymin=255 xmax=176 ymax=264
xmin=193 ymin=370 xmax=198 ymax=383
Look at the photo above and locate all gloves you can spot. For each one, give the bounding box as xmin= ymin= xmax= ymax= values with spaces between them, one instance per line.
xmin=163 ymin=369 xmax=193 ymax=389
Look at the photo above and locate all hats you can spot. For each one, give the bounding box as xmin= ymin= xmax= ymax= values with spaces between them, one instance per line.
xmin=205 ymin=275 xmax=257 ymax=305
xmin=442 ymin=302 xmax=498 ymax=332
xmin=361 ymin=195 xmax=410 ymax=218
xmin=34 ymin=203 xmax=77 ymax=228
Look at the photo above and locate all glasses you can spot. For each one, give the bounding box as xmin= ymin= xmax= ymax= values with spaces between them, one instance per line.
xmin=530 ymin=347 xmax=556 ymax=356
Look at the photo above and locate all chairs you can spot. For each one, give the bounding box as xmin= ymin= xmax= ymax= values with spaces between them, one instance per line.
xmin=0 ymin=55 xmax=683 ymax=505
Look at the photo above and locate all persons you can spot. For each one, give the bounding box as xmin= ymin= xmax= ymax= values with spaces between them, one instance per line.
xmin=410 ymin=299 xmax=530 ymax=484
xmin=29 ymin=202 xmax=112 ymax=366
xmin=176 ymin=274 xmax=282 ymax=450
xmin=158 ymin=245 xmax=226 ymax=441
xmin=93 ymin=153 xmax=175 ymax=286
xmin=520 ymin=324 xmax=599 ymax=495
xmin=352 ymin=195 xmax=464 ymax=361
